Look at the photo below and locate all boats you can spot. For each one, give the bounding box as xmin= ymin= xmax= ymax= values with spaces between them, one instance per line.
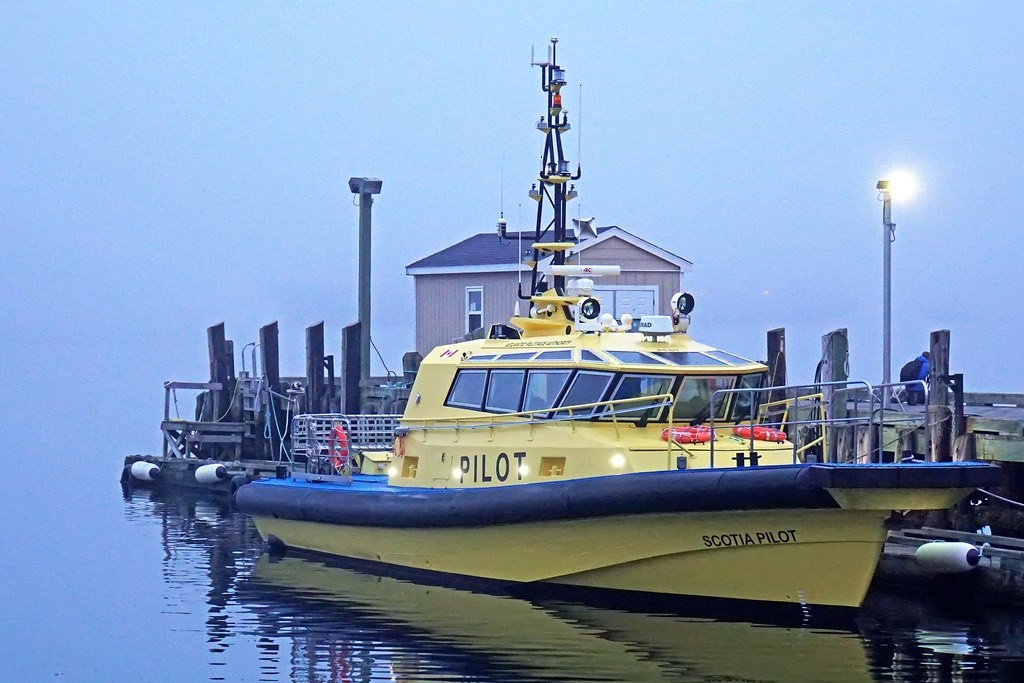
xmin=226 ymin=42 xmax=1023 ymax=616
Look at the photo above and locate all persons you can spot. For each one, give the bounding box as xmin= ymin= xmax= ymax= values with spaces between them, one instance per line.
xmin=905 ymin=351 xmax=930 ymax=405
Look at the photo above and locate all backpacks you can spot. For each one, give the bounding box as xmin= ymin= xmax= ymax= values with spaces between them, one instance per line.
xmin=900 ymin=358 xmax=927 ymax=386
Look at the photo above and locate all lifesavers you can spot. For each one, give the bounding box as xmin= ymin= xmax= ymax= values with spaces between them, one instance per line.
xmin=327 ymin=425 xmax=349 ymax=468
xmin=660 ymin=424 xmax=717 ymax=444
xmin=733 ymin=424 xmax=788 ymax=441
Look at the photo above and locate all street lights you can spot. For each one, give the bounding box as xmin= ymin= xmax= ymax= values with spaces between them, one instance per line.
xmin=348 ymin=176 xmax=381 ymax=384
xmin=874 ymin=175 xmax=913 ymax=408
